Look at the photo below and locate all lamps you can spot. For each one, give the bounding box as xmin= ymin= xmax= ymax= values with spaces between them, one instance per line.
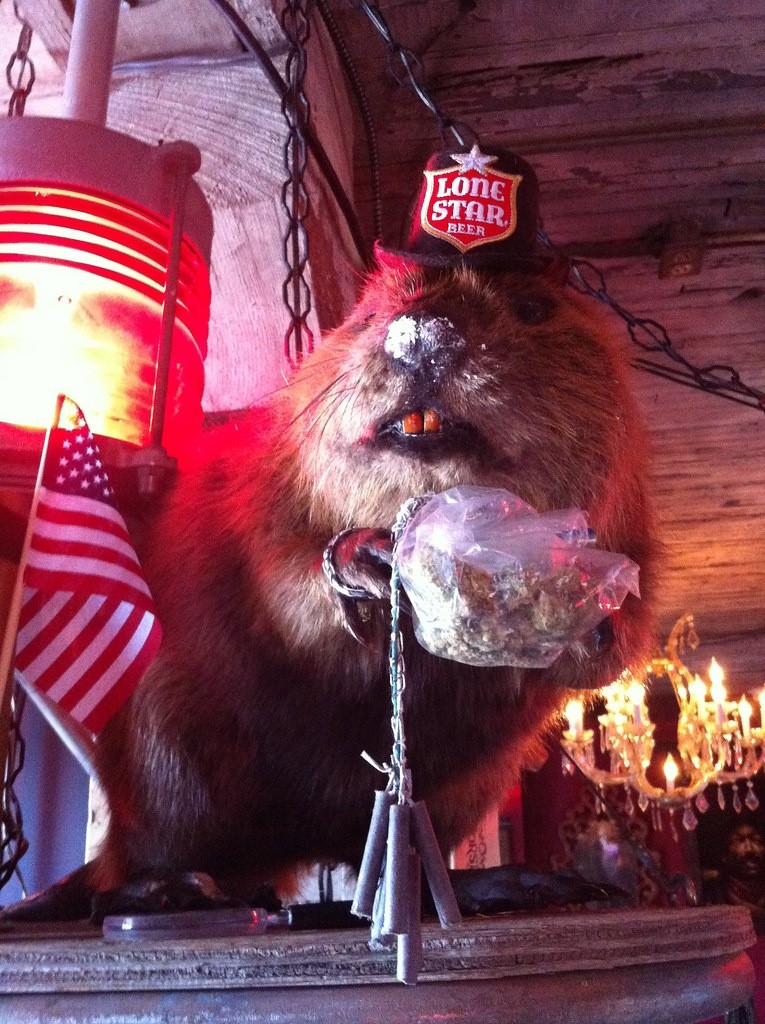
xmin=1 ymin=0 xmax=213 ymax=561
xmin=563 ymin=614 xmax=765 ymax=902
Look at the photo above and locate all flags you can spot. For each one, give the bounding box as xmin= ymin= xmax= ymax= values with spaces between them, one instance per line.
xmin=13 ymin=395 xmax=165 ymax=741
